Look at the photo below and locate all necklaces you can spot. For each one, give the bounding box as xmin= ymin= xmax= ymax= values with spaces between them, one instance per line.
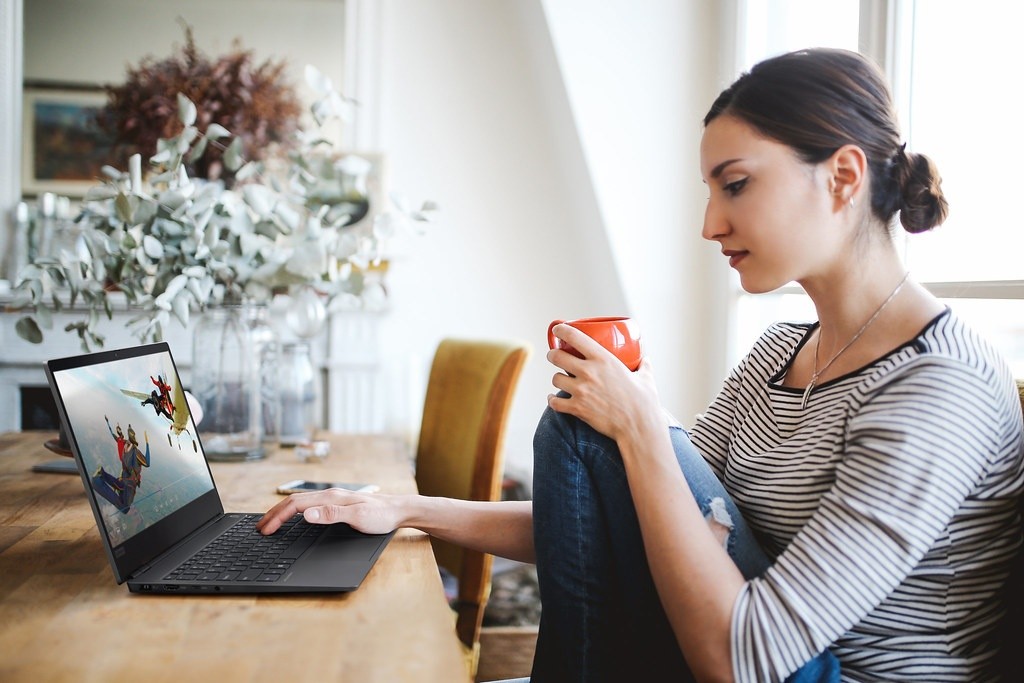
xmin=795 ymin=271 xmax=917 ymax=409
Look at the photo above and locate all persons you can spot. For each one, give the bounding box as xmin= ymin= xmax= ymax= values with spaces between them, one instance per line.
xmin=242 ymin=47 xmax=1024 ymax=683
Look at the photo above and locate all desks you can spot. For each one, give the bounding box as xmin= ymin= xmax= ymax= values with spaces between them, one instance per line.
xmin=0 ymin=430 xmax=471 ymax=683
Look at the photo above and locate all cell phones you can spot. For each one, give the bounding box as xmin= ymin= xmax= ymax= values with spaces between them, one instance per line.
xmin=277 ymin=480 xmax=376 ymax=499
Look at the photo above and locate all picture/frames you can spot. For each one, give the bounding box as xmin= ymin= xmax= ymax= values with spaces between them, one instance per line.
xmin=19 ymin=75 xmax=163 ymax=200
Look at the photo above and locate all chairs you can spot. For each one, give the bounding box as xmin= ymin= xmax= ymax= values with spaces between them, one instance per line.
xmin=411 ymin=338 xmax=528 ymax=682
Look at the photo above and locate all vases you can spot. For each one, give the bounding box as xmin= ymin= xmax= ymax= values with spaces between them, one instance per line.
xmin=190 ymin=302 xmax=269 ymax=462
xmin=270 ymin=342 xmax=324 ymax=448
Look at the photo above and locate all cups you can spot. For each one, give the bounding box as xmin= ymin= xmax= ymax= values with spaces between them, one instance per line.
xmin=548 ymin=315 xmax=642 ymax=379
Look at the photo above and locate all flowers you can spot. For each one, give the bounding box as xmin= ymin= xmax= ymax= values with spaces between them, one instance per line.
xmin=8 ymin=93 xmax=403 ymax=355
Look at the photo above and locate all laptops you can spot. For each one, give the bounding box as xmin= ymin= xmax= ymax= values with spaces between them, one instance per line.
xmin=44 ymin=340 xmax=401 ymax=595
